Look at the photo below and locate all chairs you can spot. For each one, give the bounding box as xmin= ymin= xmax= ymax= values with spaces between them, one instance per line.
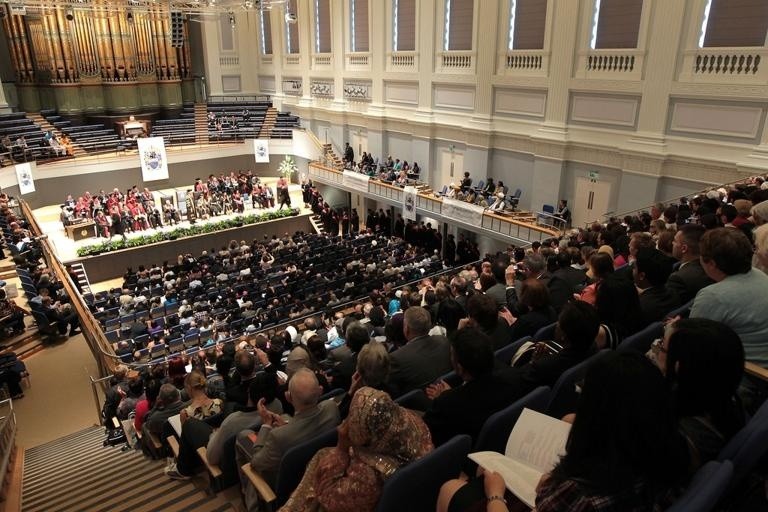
xmin=0 ymin=98 xmax=768 ymax=511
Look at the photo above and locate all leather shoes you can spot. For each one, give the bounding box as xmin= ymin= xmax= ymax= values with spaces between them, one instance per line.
xmin=69 ymin=330 xmax=81 ymax=337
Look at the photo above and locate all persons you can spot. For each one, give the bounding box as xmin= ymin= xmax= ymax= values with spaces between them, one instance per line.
xmin=386 ymin=172 xmax=408 ymax=185
xmin=60 ymin=134 xmax=73 ymax=156
xmin=438 ymin=173 xmax=506 ymax=213
xmin=582 ymin=173 xmax=768 ymax=512
xmin=207 ymin=108 xmax=239 ymax=141
xmin=0 ymin=189 xmax=83 ymax=401
xmin=15 ymin=134 xmax=27 ymax=150
xmin=84 ymin=185 xmax=178 ymax=238
xmin=301 ymin=178 xmax=340 ymax=237
xmin=342 ymin=142 xmax=419 ymax=174
xmin=553 ymin=199 xmax=571 ymax=226
xmin=2 ymin=134 xmax=12 ymax=152
xmin=236 ymin=207 xmax=615 ymax=512
xmin=186 ymin=172 xmax=291 ymax=222
xmin=86 ymin=241 xmax=282 ymax=489
xmin=49 ymin=134 xmax=66 ymax=155
xmin=242 ymin=108 xmax=250 ymax=126
xmin=45 ymin=129 xmax=55 ymax=145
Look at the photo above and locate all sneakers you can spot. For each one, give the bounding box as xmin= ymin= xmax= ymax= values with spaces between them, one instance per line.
xmin=164 ymin=462 xmax=193 ymax=480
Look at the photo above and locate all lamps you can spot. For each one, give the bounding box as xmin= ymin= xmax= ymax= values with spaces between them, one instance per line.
xmin=8 ymin=2 xmax=297 ymax=26
